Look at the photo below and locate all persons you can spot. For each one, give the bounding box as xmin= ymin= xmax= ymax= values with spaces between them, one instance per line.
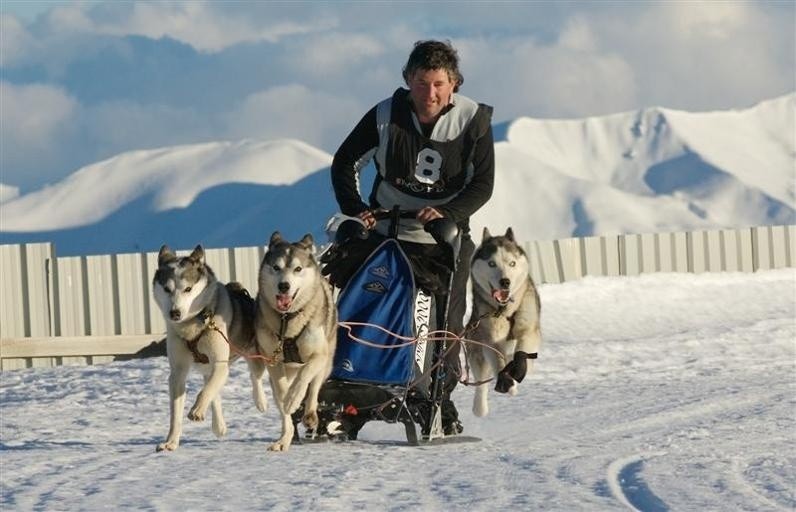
xmin=330 ymin=40 xmax=496 ymax=436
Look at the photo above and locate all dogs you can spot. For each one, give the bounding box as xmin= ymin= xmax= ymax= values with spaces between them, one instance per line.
xmin=151 ymin=242 xmax=269 ymax=451
xmin=465 ymin=226 xmax=543 ymax=418
xmin=252 ymin=230 xmax=339 ymax=453
